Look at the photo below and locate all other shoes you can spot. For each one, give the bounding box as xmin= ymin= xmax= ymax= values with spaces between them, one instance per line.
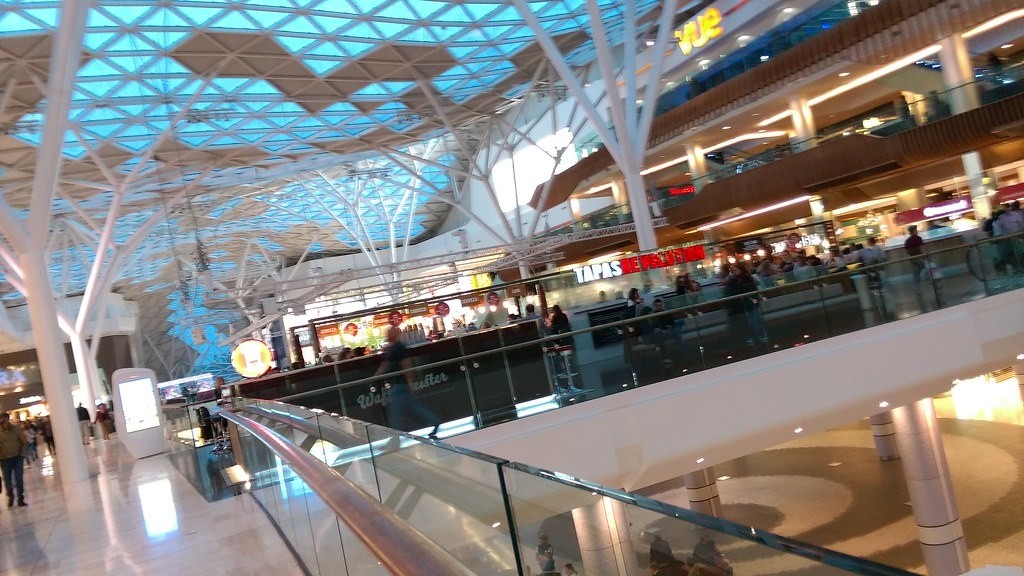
xmin=16 ymin=497 xmax=28 ymax=506
xmin=7 ymin=494 xmax=15 ymax=508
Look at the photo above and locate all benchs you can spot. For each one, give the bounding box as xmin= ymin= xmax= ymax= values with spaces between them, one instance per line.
xmin=627 ymin=280 xmax=860 ymax=359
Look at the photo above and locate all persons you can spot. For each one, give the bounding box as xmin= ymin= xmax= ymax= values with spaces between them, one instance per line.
xmin=0 ymin=413 xmax=31 ymax=508
xmin=17 ymin=417 xmax=57 ymax=466
xmin=899 ymin=95 xmax=912 ymax=118
xmin=94 ymin=404 xmax=113 ymax=442
xmin=76 ymin=402 xmax=92 ymax=446
xmin=215 ymin=190 xmax=1023 ymax=397
